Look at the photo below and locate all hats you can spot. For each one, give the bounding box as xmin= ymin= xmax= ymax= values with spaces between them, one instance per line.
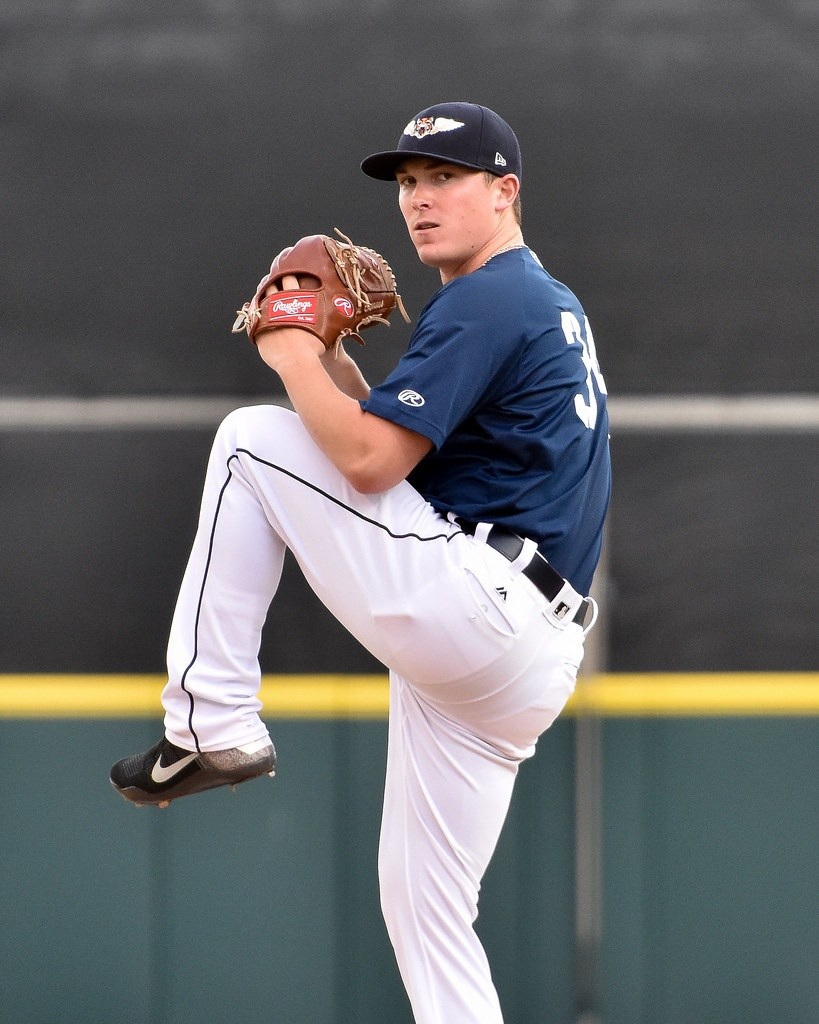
xmin=360 ymin=101 xmax=523 ymax=183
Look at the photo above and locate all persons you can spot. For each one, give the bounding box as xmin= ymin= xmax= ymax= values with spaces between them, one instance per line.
xmin=111 ymin=100 xmax=614 ymax=1024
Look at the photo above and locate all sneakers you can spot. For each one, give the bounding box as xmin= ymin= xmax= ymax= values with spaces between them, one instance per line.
xmin=108 ymin=734 xmax=278 ymax=809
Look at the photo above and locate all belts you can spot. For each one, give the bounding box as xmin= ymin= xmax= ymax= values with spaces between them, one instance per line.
xmin=465 ymin=522 xmax=588 ymax=626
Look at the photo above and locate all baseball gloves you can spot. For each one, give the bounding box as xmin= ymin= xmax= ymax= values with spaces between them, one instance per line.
xmin=245 ymin=234 xmax=398 ymax=352
